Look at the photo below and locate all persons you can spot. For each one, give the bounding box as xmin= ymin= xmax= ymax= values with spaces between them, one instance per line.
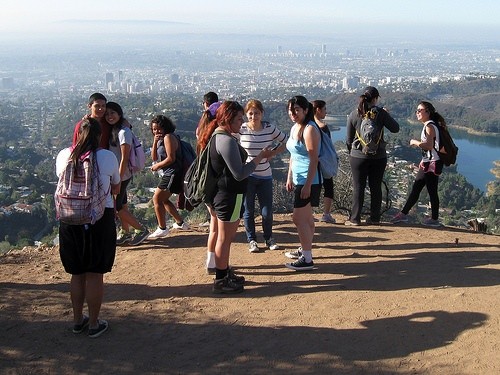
xmin=56 ymin=117 xmax=121 ymax=338
xmin=195 ymin=92 xmax=222 ymax=155
xmin=344 ymin=86 xmax=400 ymax=226
xmin=310 ymin=100 xmax=336 ymax=224
xmin=390 ymin=101 xmax=444 ymax=227
xmin=72 ymin=93 xmax=151 ymax=245
xmin=148 ymin=115 xmax=188 ymax=238
xmin=284 ymin=95 xmax=322 ymax=270
xmin=204 ymin=101 xmax=279 ymax=294
xmin=233 ymin=99 xmax=289 ymax=252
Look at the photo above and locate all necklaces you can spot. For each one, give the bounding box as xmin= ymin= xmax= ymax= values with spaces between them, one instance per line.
xmin=248 ymin=129 xmax=256 ymax=139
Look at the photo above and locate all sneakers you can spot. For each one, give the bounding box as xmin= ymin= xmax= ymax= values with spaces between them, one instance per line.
xmin=248 ymin=240 xmax=259 ymax=251
xmin=285 ymin=246 xmax=313 ymax=259
xmin=285 ymin=256 xmax=314 ymax=269
xmin=265 ymin=236 xmax=279 ymax=250
xmin=114 ymin=225 xmax=151 ymax=245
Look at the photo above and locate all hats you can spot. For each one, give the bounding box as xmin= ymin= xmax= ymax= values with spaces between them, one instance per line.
xmin=207 ymin=101 xmax=221 ymax=116
xmin=364 ymin=86 xmax=381 ymax=98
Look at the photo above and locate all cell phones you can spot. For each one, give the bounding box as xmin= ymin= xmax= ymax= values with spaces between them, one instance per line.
xmin=272 ymin=142 xmax=282 ymax=151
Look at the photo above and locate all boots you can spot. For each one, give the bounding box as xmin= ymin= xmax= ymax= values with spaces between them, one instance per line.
xmin=211 ymin=267 xmax=245 ymax=294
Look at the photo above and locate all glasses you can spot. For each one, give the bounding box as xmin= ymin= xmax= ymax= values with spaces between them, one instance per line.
xmin=414 ymin=108 xmax=425 ymax=111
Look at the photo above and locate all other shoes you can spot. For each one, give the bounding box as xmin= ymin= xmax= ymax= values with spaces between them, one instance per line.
xmin=73 ymin=313 xmax=108 ymax=337
xmin=420 ymin=217 xmax=440 ymax=226
xmin=344 ymin=217 xmax=380 ymax=226
xmin=314 ymin=212 xmax=336 ymax=223
xmin=149 ymin=226 xmax=170 ymax=238
xmin=173 ymin=222 xmax=189 ymax=230
xmin=389 ymin=212 xmax=408 ymax=222
xmin=206 ymin=266 xmax=216 ymax=274
xmin=199 ymin=221 xmax=210 ymax=226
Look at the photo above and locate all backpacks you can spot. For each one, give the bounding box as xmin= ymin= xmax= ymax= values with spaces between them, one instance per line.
xmin=53 ymin=144 xmax=111 ymax=226
xmin=161 ymin=133 xmax=195 ymax=174
xmin=299 ymin=121 xmax=339 ymax=179
xmin=194 ymin=129 xmax=249 ymax=206
xmin=117 ymin=124 xmax=145 ymax=172
xmin=184 ymin=156 xmax=206 ymax=206
xmin=423 ymin=121 xmax=457 ymax=166
xmin=352 ymin=105 xmax=384 ymax=156
